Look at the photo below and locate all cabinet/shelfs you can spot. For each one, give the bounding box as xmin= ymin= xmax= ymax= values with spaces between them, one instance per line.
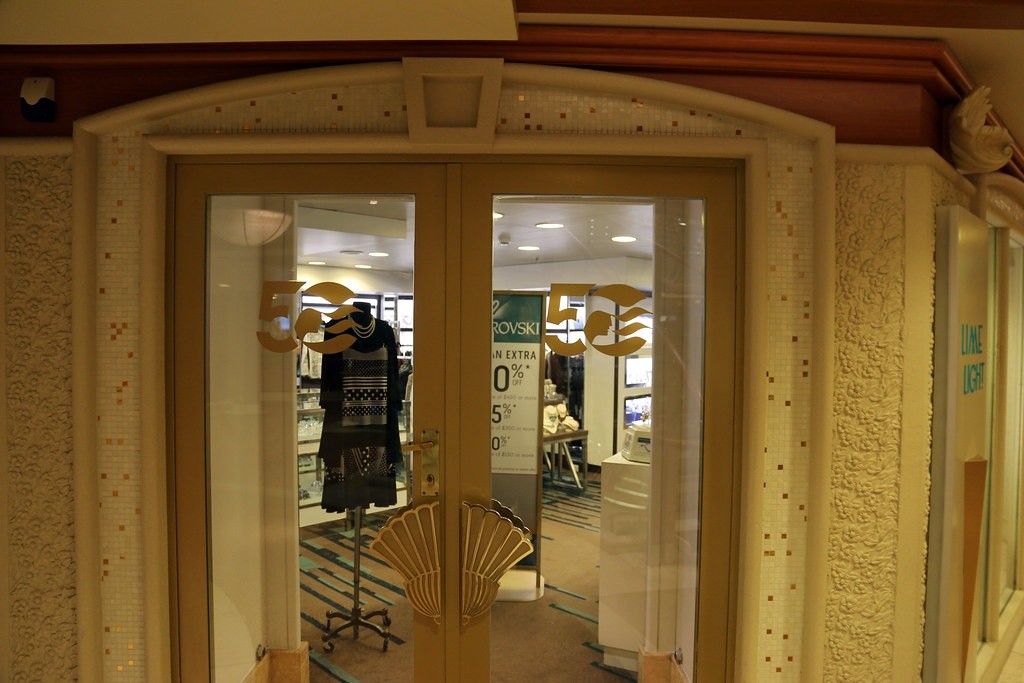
xmin=299 ymin=291 xmax=414 ymax=379
xmin=296 ymin=387 xmax=412 ymax=532
xmin=543 ymin=378 xmax=589 ymax=492
xmin=598 ymin=451 xmax=652 ymax=672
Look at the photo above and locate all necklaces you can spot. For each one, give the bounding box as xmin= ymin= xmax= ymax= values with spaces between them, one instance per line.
xmin=348 ymin=315 xmax=376 ymax=339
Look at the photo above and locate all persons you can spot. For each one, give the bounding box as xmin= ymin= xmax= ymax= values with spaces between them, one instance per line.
xmin=317 ymin=302 xmax=403 ymax=513
